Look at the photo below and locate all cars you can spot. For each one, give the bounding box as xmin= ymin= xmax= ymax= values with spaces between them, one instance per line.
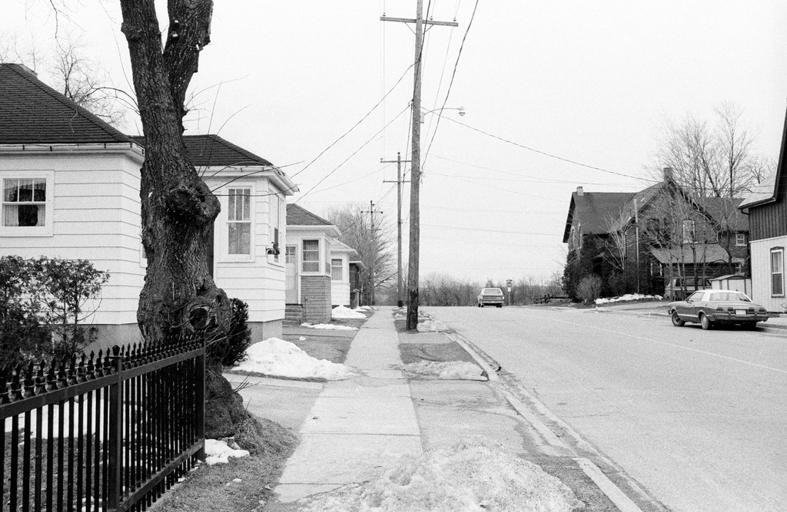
xmin=664 ymin=288 xmax=771 ymax=330
xmin=474 ymin=287 xmax=506 ymax=308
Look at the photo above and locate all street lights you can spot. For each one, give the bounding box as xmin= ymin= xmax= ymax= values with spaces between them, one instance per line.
xmin=415 ymin=106 xmax=466 ymax=122
